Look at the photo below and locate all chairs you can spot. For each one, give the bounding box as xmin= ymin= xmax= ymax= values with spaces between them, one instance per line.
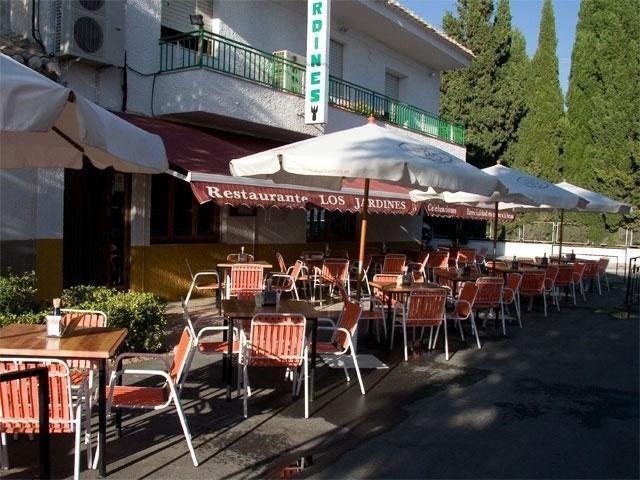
xmin=580 ymin=263 xmax=601 ymax=295
xmin=406 ymin=253 xmax=430 ymax=278
xmin=382 ymin=254 xmax=406 ymax=273
xmin=350 ymin=256 xmax=373 ymax=295
xmin=227 ymin=255 xmax=253 ymax=263
xmin=296 ymin=299 xmax=365 ymax=398
xmin=237 ymin=313 xmax=309 ymax=419
xmin=373 ymin=275 xmax=402 ymax=281
xmin=471 ymin=277 xmax=505 ymax=335
xmin=181 ymin=298 xmax=239 ymax=354
xmin=88 ymin=327 xmax=198 ymax=469
xmin=552 ymin=265 xmax=576 ymax=305
xmin=0 ymin=359 xmax=92 ymax=480
xmin=482 ymin=272 xmax=523 ymax=327
xmin=456 ymin=248 xmax=477 ymax=268
xmin=315 ymin=258 xmax=351 ymax=300
xmin=268 ymin=260 xmax=302 ymax=311
xmin=585 ymin=259 xmax=610 ymax=292
xmin=269 ymin=252 xmax=287 ymax=275
xmin=573 ymin=264 xmax=586 ymax=301
xmin=298 ymin=251 xmax=325 ymax=296
xmin=395 ymin=289 xmax=449 ymax=360
xmin=528 ymin=266 xmax=560 ymax=312
xmin=59 ymin=309 xmax=107 ymax=368
xmin=520 ymin=271 xmax=547 ymax=317
xmin=427 ymin=251 xmax=449 ymax=278
xmin=432 ymin=281 xmax=482 ymax=349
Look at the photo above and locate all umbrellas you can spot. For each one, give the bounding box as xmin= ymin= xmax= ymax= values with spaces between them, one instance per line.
xmin=1 ymin=51 xmax=170 ymax=176
xmin=408 ymin=162 xmax=591 ymax=277
xmin=227 ymin=114 xmax=498 ymax=304
xmin=496 ymin=178 xmax=633 ymax=263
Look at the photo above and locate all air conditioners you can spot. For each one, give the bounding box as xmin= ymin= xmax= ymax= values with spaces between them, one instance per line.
xmin=55 ymin=2 xmax=127 ymax=68
